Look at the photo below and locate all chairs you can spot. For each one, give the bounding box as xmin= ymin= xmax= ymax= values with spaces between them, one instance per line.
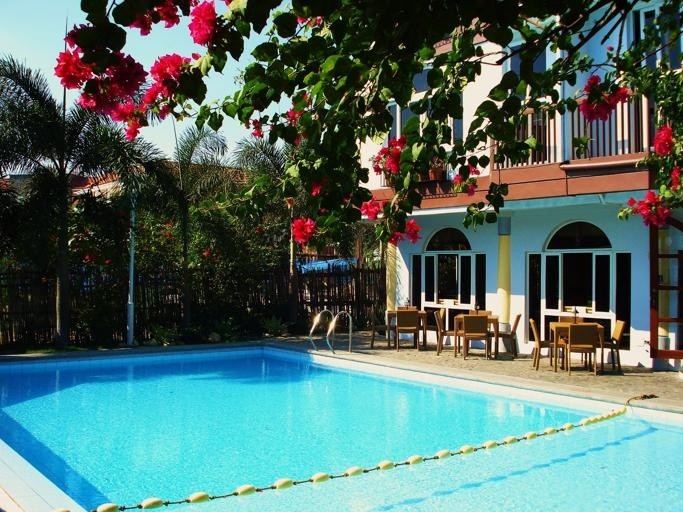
xmin=433 ymin=310 xmax=521 ymax=361
xmin=396 ymin=307 xmax=421 ymax=351
xmin=529 ymin=316 xmax=625 ymax=374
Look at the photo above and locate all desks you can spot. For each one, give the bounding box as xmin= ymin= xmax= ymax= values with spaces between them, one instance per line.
xmin=386 ymin=310 xmax=428 ymax=349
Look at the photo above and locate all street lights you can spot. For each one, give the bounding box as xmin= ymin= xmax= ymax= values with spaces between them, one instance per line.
xmin=124 ymin=165 xmax=146 ymax=347
